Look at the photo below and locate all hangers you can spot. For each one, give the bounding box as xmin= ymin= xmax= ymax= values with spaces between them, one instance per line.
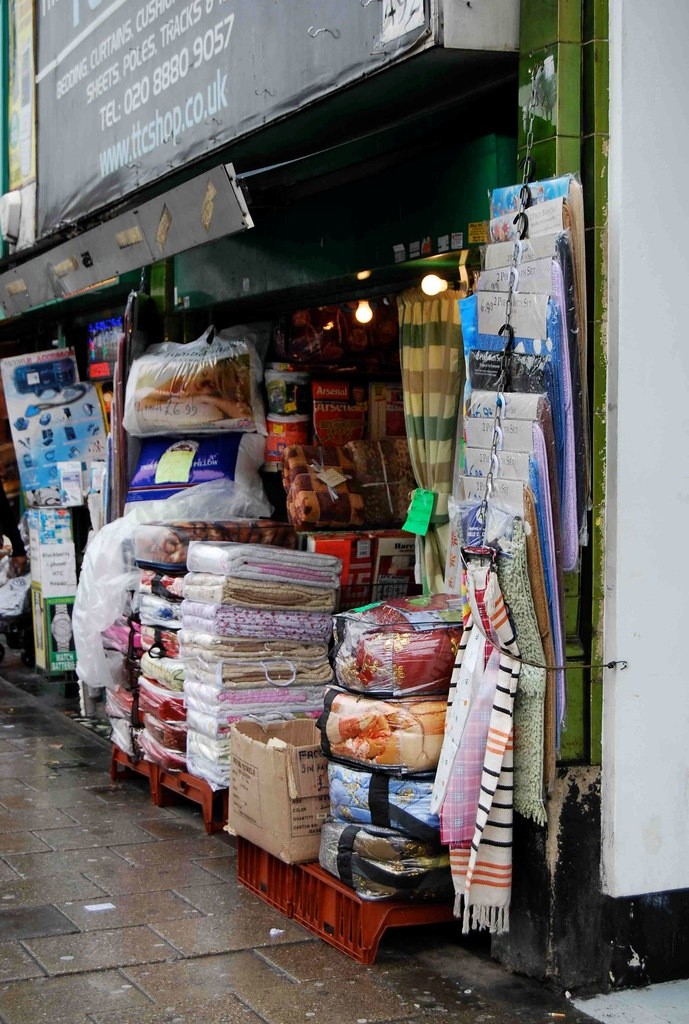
xmin=454 ymin=156 xmax=576 ymax=582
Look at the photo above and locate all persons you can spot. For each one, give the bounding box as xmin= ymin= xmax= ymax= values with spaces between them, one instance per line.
xmin=0 ymin=475 xmax=27 ymax=672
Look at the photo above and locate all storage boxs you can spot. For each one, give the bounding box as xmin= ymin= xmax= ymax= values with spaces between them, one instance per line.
xmin=221 ymin=531 xmax=465 ymax=899
